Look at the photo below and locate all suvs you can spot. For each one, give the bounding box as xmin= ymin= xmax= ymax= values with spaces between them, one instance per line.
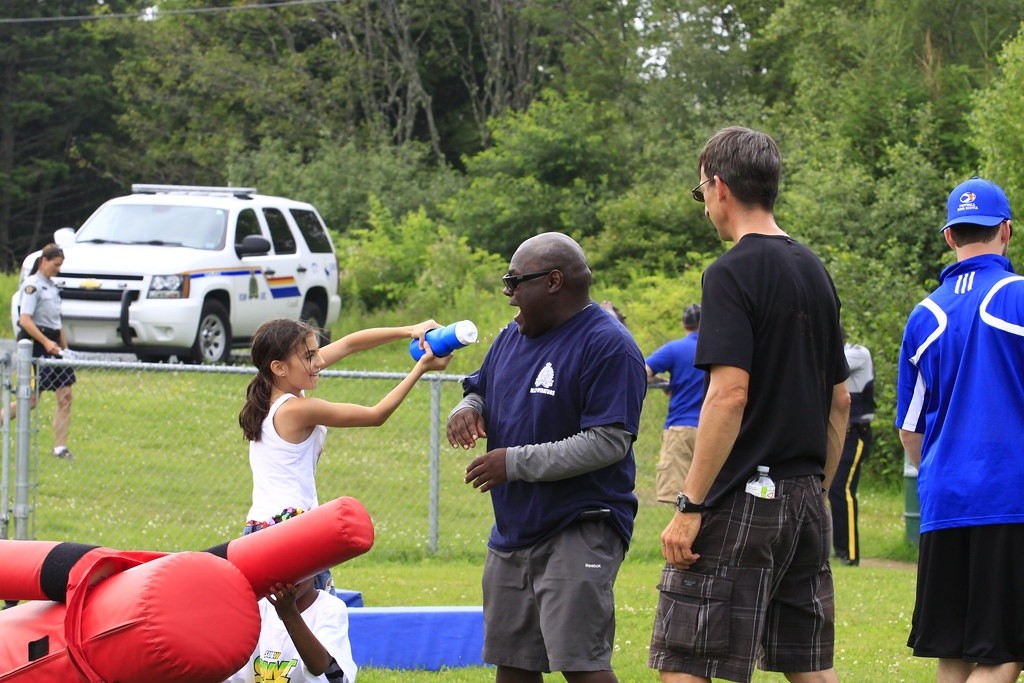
xmin=10 ymin=184 xmax=342 ymax=364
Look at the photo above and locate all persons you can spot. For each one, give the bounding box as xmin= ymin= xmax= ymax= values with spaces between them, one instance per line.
xmin=645 ymin=302 xmax=705 ymax=504
xmin=446 ymin=232 xmax=648 ymax=683
xmin=224 ymin=576 xmax=359 ymax=683
xmin=647 ymin=126 xmax=851 ymax=683
xmin=238 ymin=318 xmax=452 ymax=597
xmin=0 ymin=244 xmax=75 ymax=458
xmin=895 ymin=176 xmax=1024 ymax=683
xmin=828 ymin=326 xmax=877 ymax=568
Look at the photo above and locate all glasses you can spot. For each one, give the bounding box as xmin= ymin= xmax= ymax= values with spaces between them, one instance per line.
xmin=501 ymin=271 xmax=551 ymax=292
xmin=689 ymin=179 xmax=711 ymax=203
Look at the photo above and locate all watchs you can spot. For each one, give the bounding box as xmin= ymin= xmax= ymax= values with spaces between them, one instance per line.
xmin=675 ymin=492 xmax=705 ymax=513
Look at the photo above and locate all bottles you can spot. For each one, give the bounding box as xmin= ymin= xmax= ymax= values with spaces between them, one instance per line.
xmin=744 ymin=464 xmax=778 ymax=502
xmin=409 ymin=320 xmax=478 ymax=363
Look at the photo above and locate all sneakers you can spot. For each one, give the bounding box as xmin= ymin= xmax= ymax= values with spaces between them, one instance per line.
xmin=52 ymin=444 xmax=70 ymax=459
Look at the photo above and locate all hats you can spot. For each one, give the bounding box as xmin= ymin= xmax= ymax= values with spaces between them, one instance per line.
xmin=938 ymin=175 xmax=1013 ymax=236
